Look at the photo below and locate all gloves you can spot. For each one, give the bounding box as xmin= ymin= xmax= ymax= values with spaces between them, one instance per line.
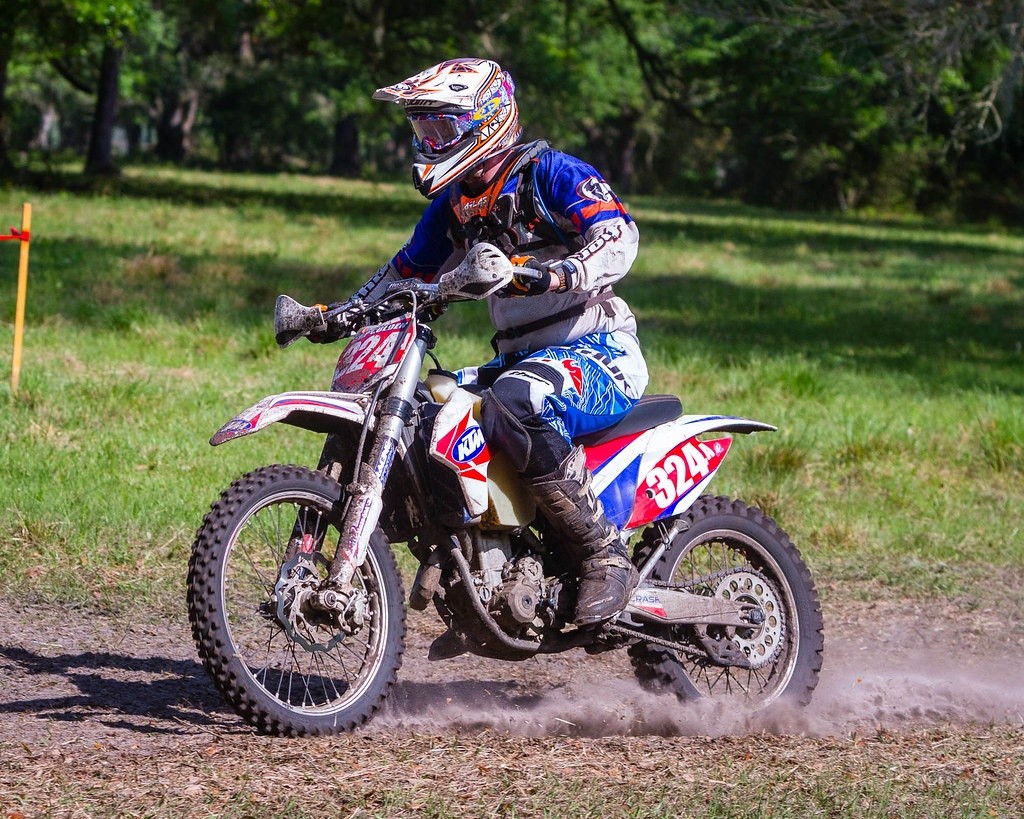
xmin=304 ymin=303 xmax=352 ymax=344
xmin=492 ymin=254 xmax=551 ymax=298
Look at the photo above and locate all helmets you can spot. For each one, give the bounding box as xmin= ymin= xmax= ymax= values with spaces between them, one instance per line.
xmin=376 ymin=57 xmax=521 ymax=200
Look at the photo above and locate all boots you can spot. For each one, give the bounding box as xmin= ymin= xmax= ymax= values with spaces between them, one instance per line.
xmin=531 ymin=442 xmax=642 ymax=626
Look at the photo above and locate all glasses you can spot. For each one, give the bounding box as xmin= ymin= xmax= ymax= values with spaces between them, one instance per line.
xmin=408 ymin=116 xmax=470 ymax=151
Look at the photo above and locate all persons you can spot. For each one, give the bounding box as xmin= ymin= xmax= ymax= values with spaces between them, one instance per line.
xmin=29 ymin=100 xmax=63 ymax=175
xmin=273 ymin=56 xmax=651 ymax=663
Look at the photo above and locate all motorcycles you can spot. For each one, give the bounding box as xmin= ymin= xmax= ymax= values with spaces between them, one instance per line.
xmin=186 ymin=243 xmax=824 ymax=737
